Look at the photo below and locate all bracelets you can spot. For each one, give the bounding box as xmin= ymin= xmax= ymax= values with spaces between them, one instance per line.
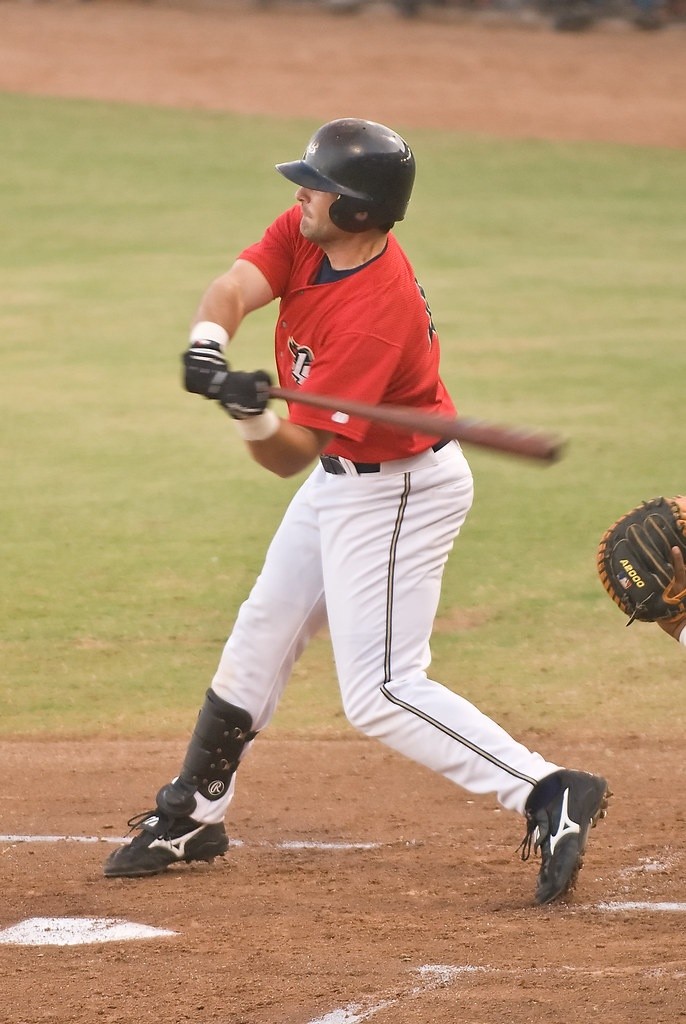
xmin=190 ymin=321 xmax=227 ymax=351
xmin=234 ymin=409 xmax=279 ymax=440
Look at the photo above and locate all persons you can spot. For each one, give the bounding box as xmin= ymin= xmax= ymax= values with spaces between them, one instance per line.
xmin=598 ymin=497 xmax=686 ymax=647
xmin=101 ymin=115 xmax=611 ymax=904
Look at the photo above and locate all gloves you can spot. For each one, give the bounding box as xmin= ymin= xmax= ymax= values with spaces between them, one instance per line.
xmin=218 ymin=370 xmax=272 ymax=421
xmin=182 ymin=340 xmax=227 ymax=393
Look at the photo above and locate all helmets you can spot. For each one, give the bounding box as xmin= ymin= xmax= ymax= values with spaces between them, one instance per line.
xmin=275 ymin=117 xmax=416 ymax=234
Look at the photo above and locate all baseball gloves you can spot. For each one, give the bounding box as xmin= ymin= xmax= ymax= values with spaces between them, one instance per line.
xmin=588 ymin=487 xmax=686 ymax=646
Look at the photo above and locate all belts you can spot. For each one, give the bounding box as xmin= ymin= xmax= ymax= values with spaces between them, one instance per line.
xmin=320 ymin=438 xmax=453 ymax=475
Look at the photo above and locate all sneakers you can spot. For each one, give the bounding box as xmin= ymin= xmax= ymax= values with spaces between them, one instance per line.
xmin=515 ymin=769 xmax=613 ymax=904
xmin=102 ymin=805 xmax=230 ymax=877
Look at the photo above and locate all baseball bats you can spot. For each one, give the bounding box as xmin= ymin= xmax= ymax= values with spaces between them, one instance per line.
xmin=250 ymin=382 xmax=578 ymax=473
